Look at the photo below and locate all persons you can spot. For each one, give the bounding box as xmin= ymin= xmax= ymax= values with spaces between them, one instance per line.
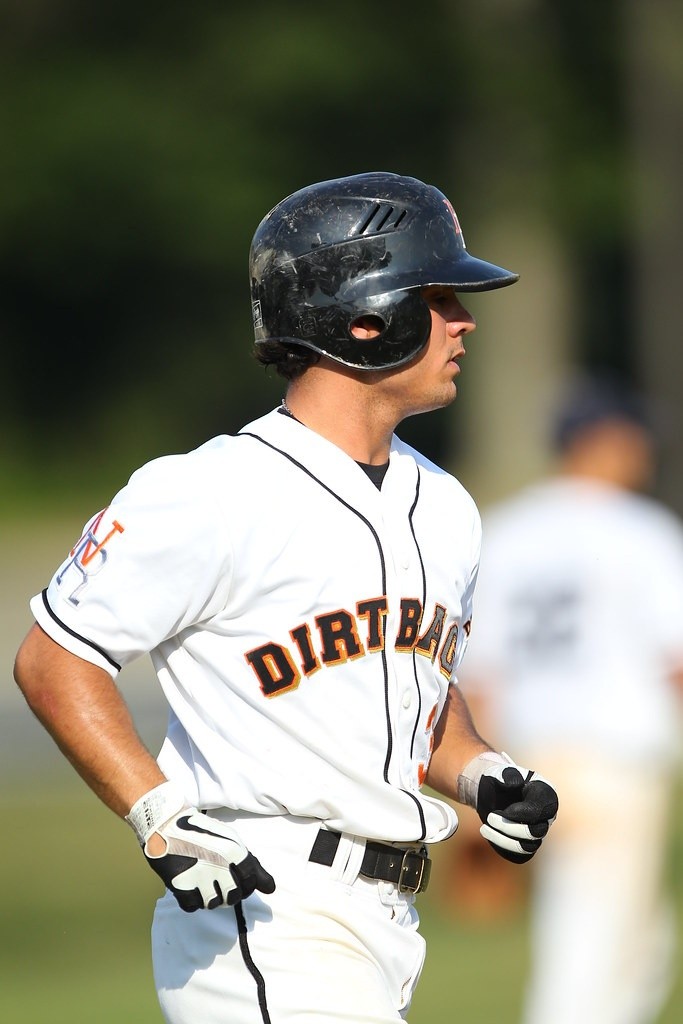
xmin=430 ymin=406 xmax=683 ymax=1024
xmin=14 ymin=171 xmax=559 ymax=1024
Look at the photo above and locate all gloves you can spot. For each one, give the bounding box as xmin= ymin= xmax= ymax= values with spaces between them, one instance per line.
xmin=122 ymin=780 xmax=276 ymax=914
xmin=456 ymin=751 xmax=559 ymax=865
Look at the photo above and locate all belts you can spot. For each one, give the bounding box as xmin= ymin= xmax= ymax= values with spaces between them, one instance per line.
xmin=308 ymin=828 xmax=432 ymax=894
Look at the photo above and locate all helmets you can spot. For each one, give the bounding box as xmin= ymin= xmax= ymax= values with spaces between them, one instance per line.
xmin=246 ymin=172 xmax=520 ymax=372
